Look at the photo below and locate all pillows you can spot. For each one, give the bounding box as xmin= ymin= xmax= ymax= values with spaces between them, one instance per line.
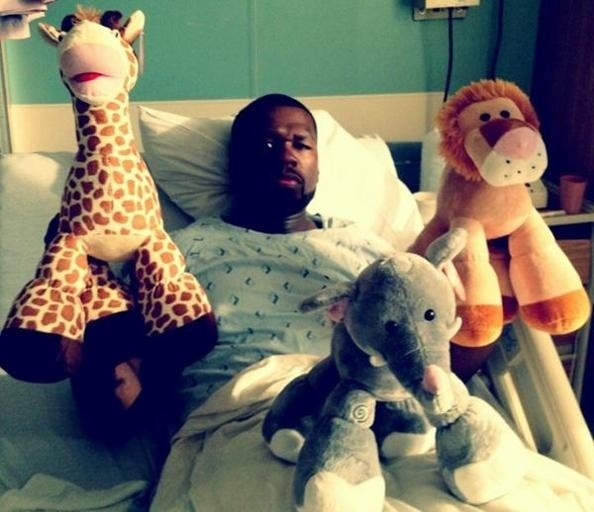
xmin=135 ymin=105 xmax=422 ymax=233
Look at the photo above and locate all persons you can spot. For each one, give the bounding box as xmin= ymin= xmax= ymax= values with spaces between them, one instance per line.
xmin=40 ymin=90 xmax=502 ymax=427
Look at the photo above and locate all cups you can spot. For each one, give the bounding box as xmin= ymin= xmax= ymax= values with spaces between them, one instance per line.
xmin=559 ymin=175 xmax=588 ymax=214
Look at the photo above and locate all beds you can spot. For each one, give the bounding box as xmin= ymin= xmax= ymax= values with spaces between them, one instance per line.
xmin=1 ymin=95 xmax=594 ymax=511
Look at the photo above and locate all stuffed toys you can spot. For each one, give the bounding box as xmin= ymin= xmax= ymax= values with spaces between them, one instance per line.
xmin=261 ymin=227 xmax=525 ymax=512
xmin=0 ymin=0 xmax=222 ymax=386
xmin=402 ymin=74 xmax=593 ymax=352
